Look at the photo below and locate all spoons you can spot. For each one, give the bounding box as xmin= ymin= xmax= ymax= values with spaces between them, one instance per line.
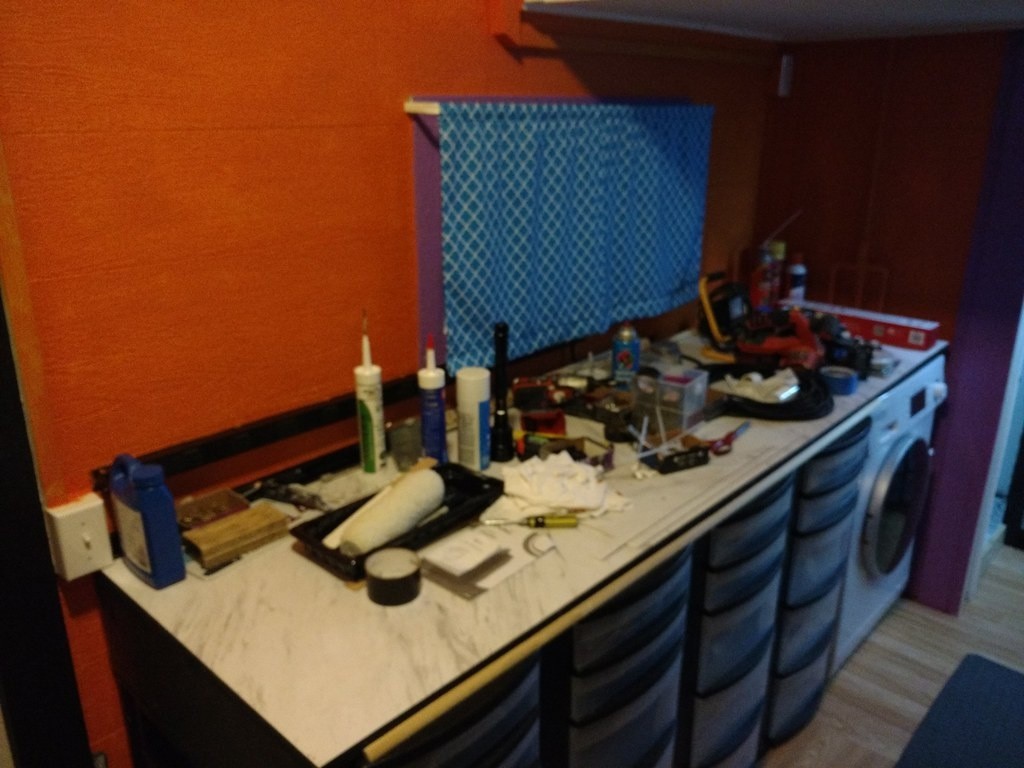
xmin=712 ymin=422 xmax=752 ymax=456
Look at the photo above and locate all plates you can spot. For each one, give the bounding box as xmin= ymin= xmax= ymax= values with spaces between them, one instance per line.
xmin=288 ymin=462 xmax=504 ymax=582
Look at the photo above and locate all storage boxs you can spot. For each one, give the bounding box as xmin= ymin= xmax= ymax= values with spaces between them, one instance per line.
xmin=818 ymin=304 xmax=941 ymax=350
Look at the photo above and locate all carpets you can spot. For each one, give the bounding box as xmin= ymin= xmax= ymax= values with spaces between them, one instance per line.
xmin=892 ymin=653 xmax=1024 ymax=768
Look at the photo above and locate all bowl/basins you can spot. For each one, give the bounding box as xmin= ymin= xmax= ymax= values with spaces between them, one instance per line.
xmin=365 ymin=548 xmax=421 ymax=606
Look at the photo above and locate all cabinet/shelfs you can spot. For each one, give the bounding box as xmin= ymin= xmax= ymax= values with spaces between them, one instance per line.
xmin=366 ymin=416 xmax=874 ymax=768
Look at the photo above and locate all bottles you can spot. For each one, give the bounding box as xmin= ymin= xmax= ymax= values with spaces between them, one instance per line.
xmin=416 ymin=332 xmax=449 ymax=464
xmin=454 ymin=367 xmax=491 ymax=472
xmin=768 ymin=242 xmax=787 ymax=300
xmin=784 ymin=252 xmax=806 ymax=300
xmin=353 ymin=335 xmax=387 ymax=472
xmin=611 ymin=325 xmax=640 ymax=384
xmin=109 ymin=452 xmax=186 ymax=590
